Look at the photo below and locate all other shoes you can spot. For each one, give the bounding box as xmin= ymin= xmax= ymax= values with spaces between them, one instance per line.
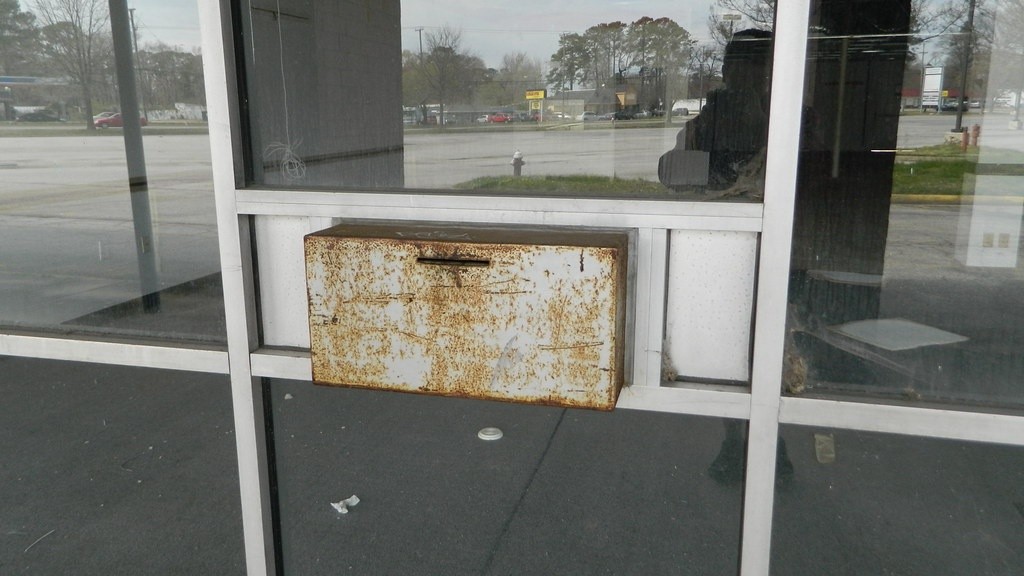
xmin=776 ymin=436 xmax=793 ymax=478
xmin=708 ymin=438 xmax=745 ymax=480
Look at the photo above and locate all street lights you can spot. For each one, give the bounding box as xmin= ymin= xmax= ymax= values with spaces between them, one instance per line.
xmin=127 ymin=8 xmax=147 ymax=123
xmin=415 ymin=28 xmax=425 ymax=55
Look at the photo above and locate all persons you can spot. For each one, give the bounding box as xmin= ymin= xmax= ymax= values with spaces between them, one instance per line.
xmin=663 ymin=27 xmax=824 ymax=485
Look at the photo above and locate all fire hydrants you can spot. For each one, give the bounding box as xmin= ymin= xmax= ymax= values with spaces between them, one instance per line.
xmin=972 ymin=124 xmax=980 ymax=146
xmin=510 ymin=151 xmax=526 ymax=175
xmin=962 ymin=126 xmax=969 ymax=151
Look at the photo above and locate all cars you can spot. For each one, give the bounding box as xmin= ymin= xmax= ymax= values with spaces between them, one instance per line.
xmin=634 ymin=108 xmax=650 ymax=118
xmin=489 ymin=112 xmax=512 ymax=124
xmin=477 ymin=115 xmax=489 ymax=124
xmin=93 ymin=112 xmax=114 ymax=120
xmin=605 ymin=111 xmax=633 ymax=120
xmin=94 ymin=113 xmax=146 ymax=129
xmin=582 ymin=111 xmax=599 ymax=121
